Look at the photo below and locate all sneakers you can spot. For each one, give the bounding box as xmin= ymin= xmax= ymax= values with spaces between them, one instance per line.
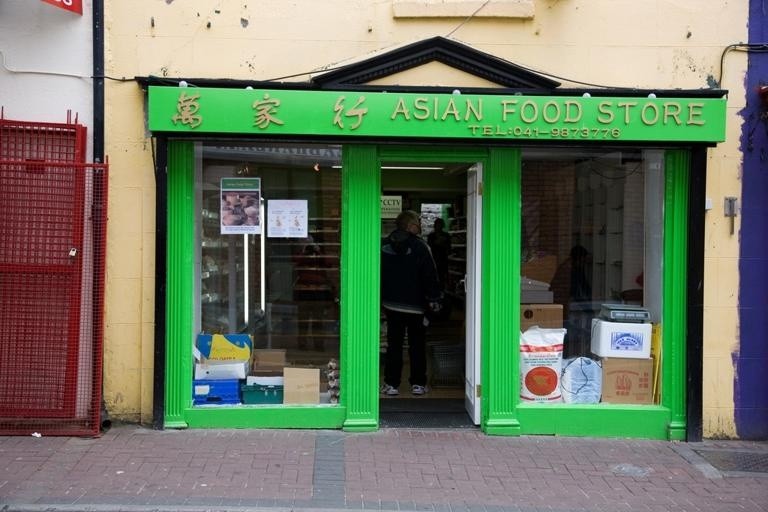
xmin=379 ymin=383 xmax=401 ymax=396
xmin=411 ymin=385 xmax=432 ymax=395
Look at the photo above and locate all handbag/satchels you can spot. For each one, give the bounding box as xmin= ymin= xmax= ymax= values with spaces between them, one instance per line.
xmin=424 ymin=291 xmax=452 ymax=320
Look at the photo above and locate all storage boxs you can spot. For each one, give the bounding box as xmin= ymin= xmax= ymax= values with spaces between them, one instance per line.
xmin=519 ymin=250 xmax=557 ymax=285
xmin=240 ymin=348 xmax=321 ymax=405
xmin=519 ymin=302 xmax=564 ymax=332
xmin=589 ymin=316 xmax=654 ymax=404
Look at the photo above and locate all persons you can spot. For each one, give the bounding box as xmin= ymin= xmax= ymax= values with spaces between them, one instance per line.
xmin=291 ymin=233 xmax=337 ymax=352
xmin=379 ymin=209 xmax=444 ymax=396
xmin=426 ymin=218 xmax=452 ymax=289
xmin=543 ymin=243 xmax=593 ymax=312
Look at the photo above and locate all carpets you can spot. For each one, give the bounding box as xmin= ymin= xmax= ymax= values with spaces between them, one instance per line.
xmin=378 ymin=396 xmax=466 ymax=413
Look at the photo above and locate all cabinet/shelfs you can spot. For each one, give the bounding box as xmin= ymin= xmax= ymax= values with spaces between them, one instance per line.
xmin=443 ymin=216 xmax=467 ymax=301
xmin=200 ymin=209 xmax=268 ymax=338
xmin=268 ymin=216 xmax=340 ymax=339
xmin=573 ymin=153 xmax=646 ymax=307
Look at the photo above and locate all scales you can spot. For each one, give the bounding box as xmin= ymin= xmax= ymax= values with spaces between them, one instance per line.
xmin=601 ymin=303 xmax=651 ymax=323
